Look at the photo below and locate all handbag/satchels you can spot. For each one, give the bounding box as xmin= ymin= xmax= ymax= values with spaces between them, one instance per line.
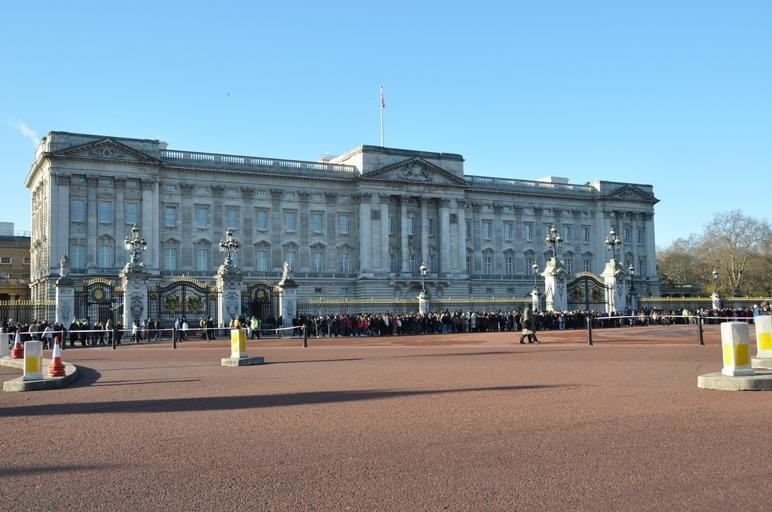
xmin=522 ymin=320 xmax=529 ymax=329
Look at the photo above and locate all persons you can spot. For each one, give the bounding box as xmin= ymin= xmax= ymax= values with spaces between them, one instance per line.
xmin=0 ymin=302 xmax=772 ymax=345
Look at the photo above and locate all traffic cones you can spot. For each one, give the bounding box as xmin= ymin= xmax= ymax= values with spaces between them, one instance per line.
xmin=11 ymin=329 xmax=24 ymax=358
xmin=47 ymin=336 xmax=66 ymax=377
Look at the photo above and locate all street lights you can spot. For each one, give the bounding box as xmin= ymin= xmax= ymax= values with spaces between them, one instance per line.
xmin=628 ymin=263 xmax=636 ymax=291
xmin=531 ymin=261 xmax=539 ymax=291
xmin=125 ymin=223 xmax=146 ymax=265
xmin=420 ymin=262 xmax=427 ymax=292
xmin=219 ymin=228 xmax=241 ymax=265
xmin=605 ymin=227 xmax=621 ymax=261
xmin=711 ymin=269 xmax=719 ymax=294
xmin=544 ymin=224 xmax=563 ymax=257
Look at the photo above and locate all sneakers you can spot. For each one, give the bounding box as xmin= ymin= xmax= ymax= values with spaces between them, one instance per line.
xmin=520 ymin=340 xmax=539 ymax=344
